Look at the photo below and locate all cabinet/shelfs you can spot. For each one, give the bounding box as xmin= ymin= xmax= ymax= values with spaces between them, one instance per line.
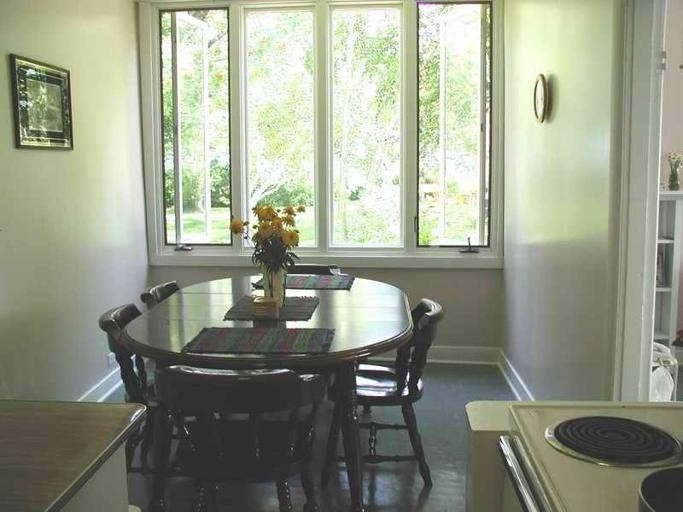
xmin=656 ymin=190 xmax=682 ymax=347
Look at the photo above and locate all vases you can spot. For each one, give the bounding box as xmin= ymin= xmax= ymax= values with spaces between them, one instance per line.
xmin=669 ymin=172 xmax=679 ymax=189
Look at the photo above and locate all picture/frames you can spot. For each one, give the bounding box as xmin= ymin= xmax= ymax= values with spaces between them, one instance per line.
xmin=9 ymin=53 xmax=74 ymax=151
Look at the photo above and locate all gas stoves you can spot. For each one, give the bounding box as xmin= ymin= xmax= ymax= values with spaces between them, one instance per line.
xmin=521 ymin=402 xmax=682 ymax=512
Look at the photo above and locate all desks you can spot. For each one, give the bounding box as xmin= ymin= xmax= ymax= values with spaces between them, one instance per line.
xmin=121 ymin=273 xmax=414 ymax=510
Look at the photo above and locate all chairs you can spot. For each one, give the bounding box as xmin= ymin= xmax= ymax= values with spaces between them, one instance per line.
xmin=139 ymin=280 xmax=178 ymax=310
xmin=158 ymin=365 xmax=318 ymax=510
xmin=291 ymin=265 xmax=338 ymax=274
xmin=318 ymin=299 xmax=443 ymax=490
xmin=100 ymin=302 xmax=165 ymax=476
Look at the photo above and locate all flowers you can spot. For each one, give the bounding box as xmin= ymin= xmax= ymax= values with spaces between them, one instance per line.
xmin=665 ymin=152 xmax=683 ymax=190
xmin=230 ymin=199 xmax=306 ymax=274
